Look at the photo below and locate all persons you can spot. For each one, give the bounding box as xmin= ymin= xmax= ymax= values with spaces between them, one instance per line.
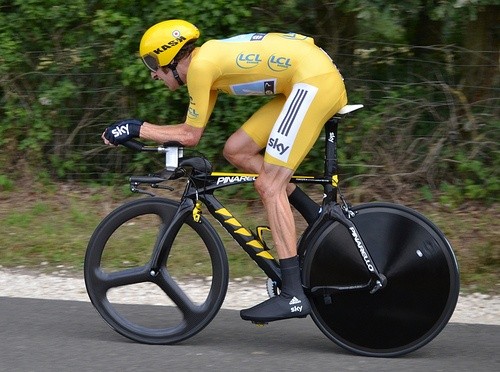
xmin=102 ymin=19 xmax=348 ymax=322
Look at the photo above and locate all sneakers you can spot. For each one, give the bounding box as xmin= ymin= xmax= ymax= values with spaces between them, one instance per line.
xmin=240 ymin=295 xmax=310 ymax=321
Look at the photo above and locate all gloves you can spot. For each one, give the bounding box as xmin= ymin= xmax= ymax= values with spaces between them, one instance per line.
xmin=103 ymin=122 xmax=147 ymax=146
xmin=140 ymin=18 xmax=201 ymax=67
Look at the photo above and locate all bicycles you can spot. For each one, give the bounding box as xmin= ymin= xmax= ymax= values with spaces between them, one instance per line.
xmin=84 ymin=104 xmax=459 ymax=357
